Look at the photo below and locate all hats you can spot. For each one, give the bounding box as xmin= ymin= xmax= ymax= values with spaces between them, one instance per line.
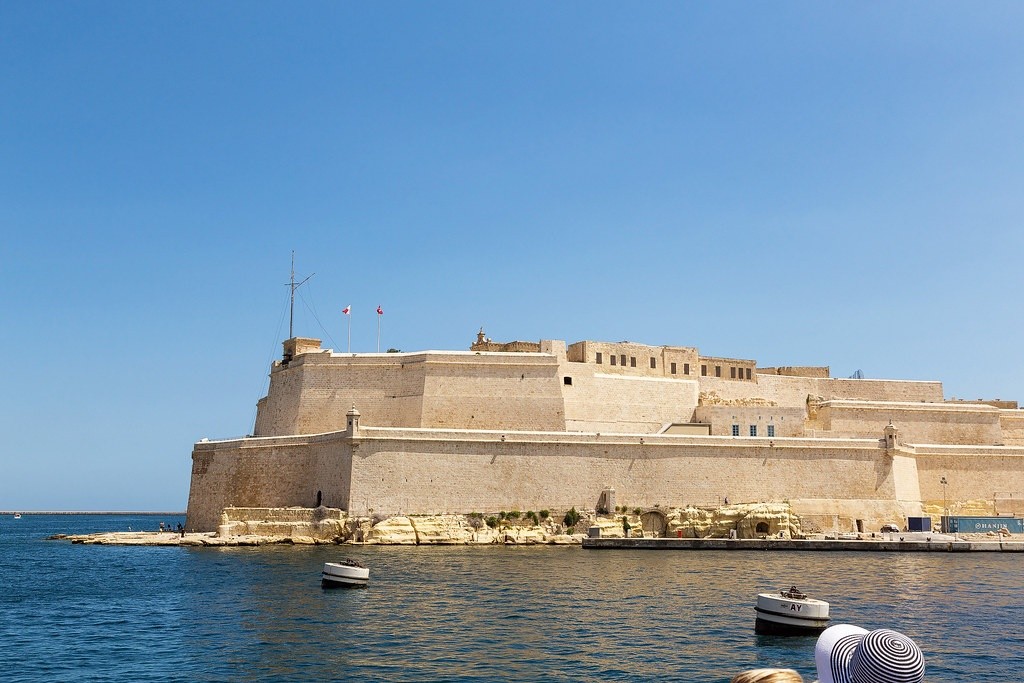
xmin=814 ymin=624 xmax=926 ymax=683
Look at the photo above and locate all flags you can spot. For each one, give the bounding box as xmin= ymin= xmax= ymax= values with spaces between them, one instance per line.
xmin=377 ymin=308 xmax=384 ymax=315
xmin=342 ymin=305 xmax=350 ymax=314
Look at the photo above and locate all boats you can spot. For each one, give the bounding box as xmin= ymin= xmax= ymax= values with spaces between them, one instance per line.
xmin=13 ymin=513 xmax=21 ymax=519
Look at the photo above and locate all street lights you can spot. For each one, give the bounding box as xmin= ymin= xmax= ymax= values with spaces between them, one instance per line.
xmin=939 ymin=476 xmax=954 ymax=533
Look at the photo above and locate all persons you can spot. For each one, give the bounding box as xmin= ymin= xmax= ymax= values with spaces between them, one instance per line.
xmin=160 ymin=521 xmax=186 ymax=539
xmin=725 ymin=497 xmax=728 ymax=505
xmin=815 ymin=623 xmax=925 ymax=683
